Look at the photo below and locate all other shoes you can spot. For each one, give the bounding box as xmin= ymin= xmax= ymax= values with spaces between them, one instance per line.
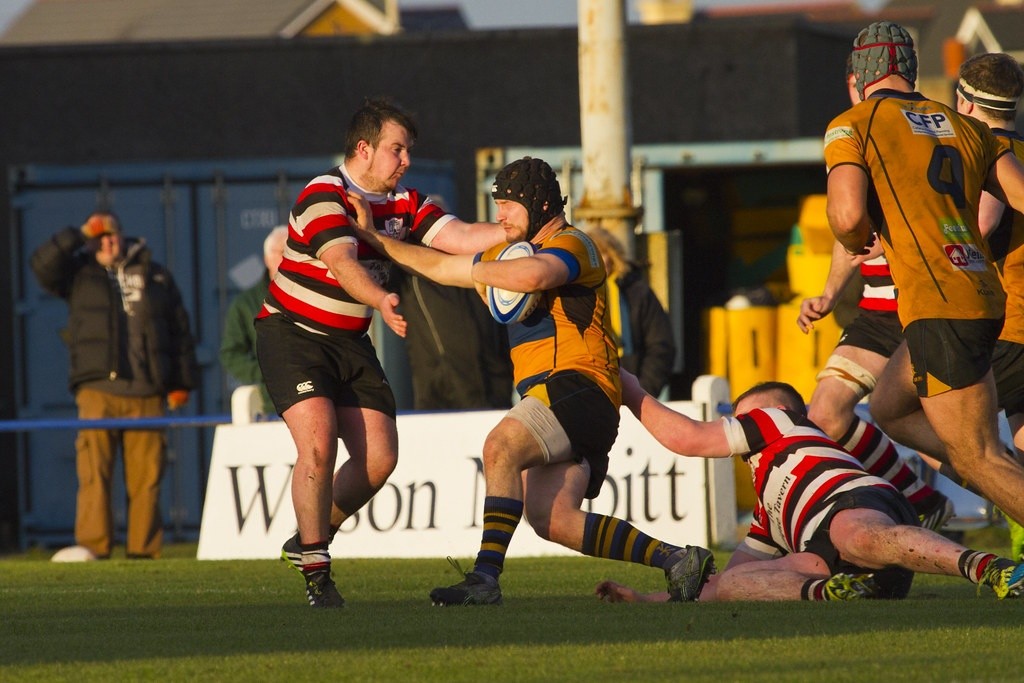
xmin=126 ymin=549 xmax=157 ymax=561
xmin=94 ymin=552 xmax=112 ymax=560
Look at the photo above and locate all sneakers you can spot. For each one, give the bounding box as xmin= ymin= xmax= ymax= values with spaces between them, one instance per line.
xmin=916 ymin=491 xmax=956 ymax=532
xmin=665 ymin=543 xmax=722 ymax=604
xmin=279 ymin=526 xmax=348 ymax=609
xmin=823 ymin=571 xmax=879 ymax=603
xmin=430 ymin=555 xmax=503 ymax=607
xmin=977 ymin=557 xmax=1023 ymax=601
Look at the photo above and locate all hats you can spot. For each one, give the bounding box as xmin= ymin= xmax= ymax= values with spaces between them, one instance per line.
xmin=491 ymin=154 xmax=568 ymax=244
xmin=851 ymin=19 xmax=920 ymax=101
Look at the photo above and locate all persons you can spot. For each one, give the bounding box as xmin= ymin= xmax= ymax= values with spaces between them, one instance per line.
xmin=254 ymin=101 xmax=506 ymax=608
xmin=724 ymin=19 xmax=1024 ymax=544
xmin=347 ymin=156 xmax=716 ymax=606
xmin=221 ymin=227 xmax=287 ymax=421
xmin=398 ymin=194 xmax=513 ymax=409
xmin=31 ymin=210 xmax=190 ymax=563
xmin=586 ymin=230 xmax=678 ymax=399
xmin=595 ymin=365 xmax=1024 ymax=603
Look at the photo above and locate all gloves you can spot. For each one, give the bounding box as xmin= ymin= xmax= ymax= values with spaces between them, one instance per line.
xmin=80 ymin=216 xmax=119 ymax=240
xmin=166 ymin=389 xmax=189 ymax=409
